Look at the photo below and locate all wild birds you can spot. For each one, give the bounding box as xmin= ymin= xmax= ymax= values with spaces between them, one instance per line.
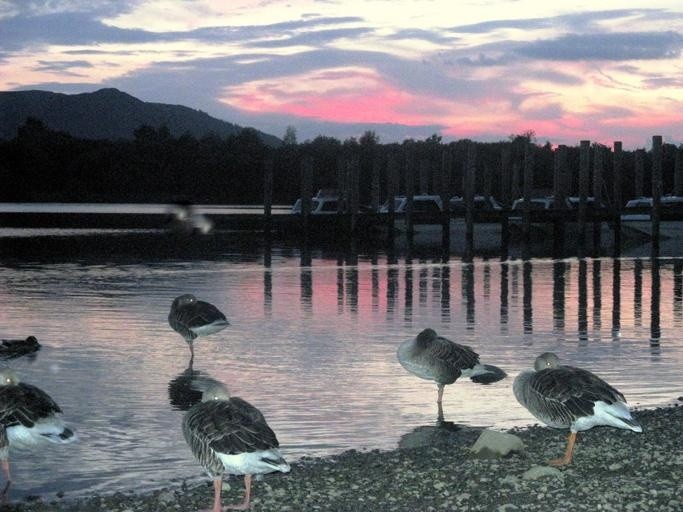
xmin=0 ymin=335 xmax=41 ymax=362
xmin=178 ymin=374 xmax=292 ymax=512
xmin=513 ymin=351 xmax=645 ymax=468
xmin=167 ymin=292 xmax=231 ymax=360
xmin=396 ymin=326 xmax=508 ymax=418
xmin=0 ymin=368 xmax=78 ymax=486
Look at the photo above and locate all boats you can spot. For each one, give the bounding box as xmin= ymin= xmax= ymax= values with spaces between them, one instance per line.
xmin=289 ymin=196 xmax=364 ymax=215
xmin=377 ymin=194 xmax=683 ymax=214
